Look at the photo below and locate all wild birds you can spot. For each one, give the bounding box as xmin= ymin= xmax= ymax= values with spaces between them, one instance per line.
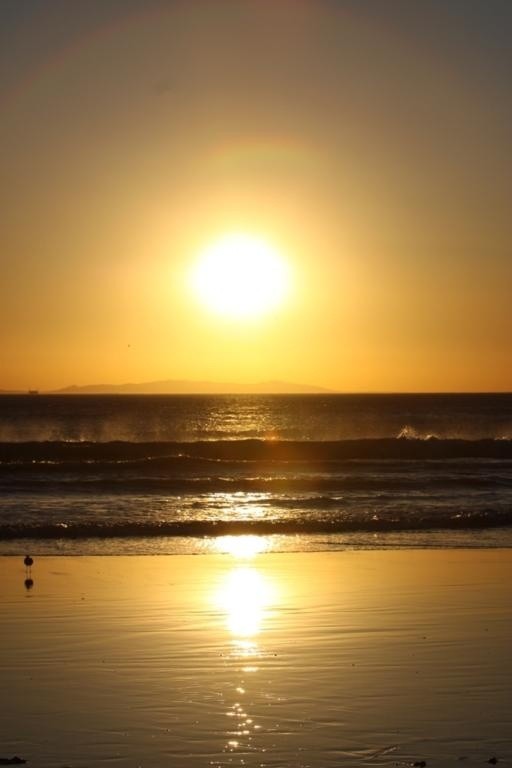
xmin=24 ymin=554 xmax=33 ymax=576
